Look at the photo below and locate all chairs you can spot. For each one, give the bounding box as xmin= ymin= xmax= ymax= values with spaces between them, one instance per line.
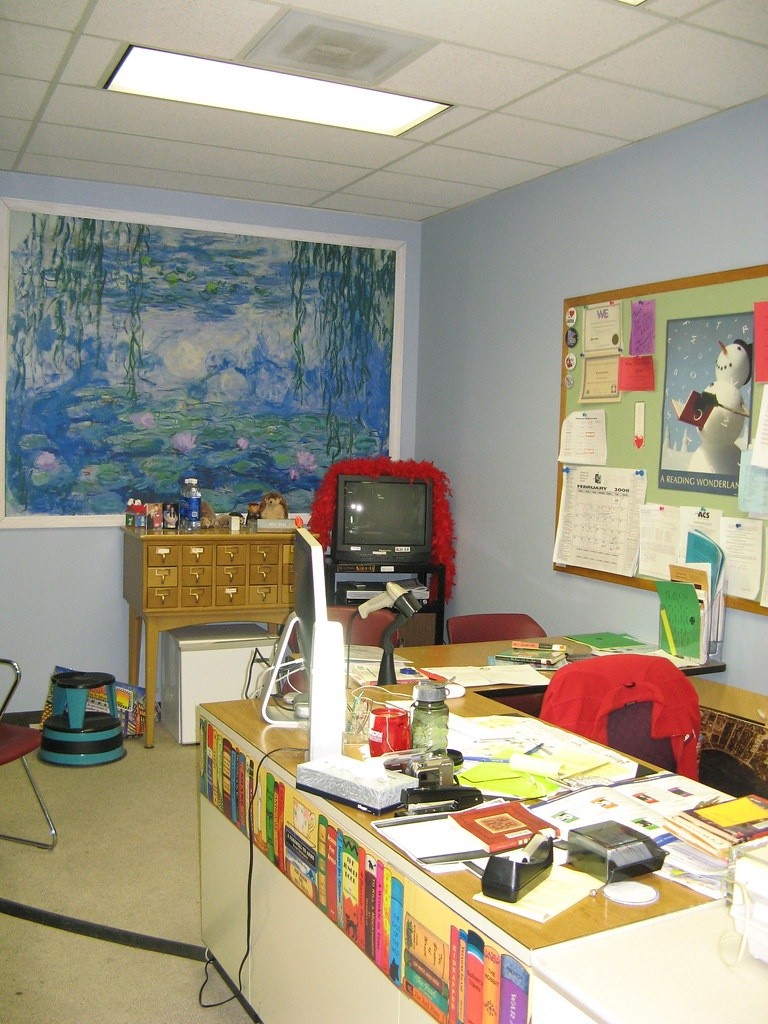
xmin=538 ymin=653 xmax=702 ymax=783
xmin=0 ymin=659 xmax=58 ymax=850
xmin=446 ymin=614 xmax=547 ymax=644
xmin=288 ymin=606 xmax=400 ymax=654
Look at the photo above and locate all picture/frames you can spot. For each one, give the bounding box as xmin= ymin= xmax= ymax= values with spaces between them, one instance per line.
xmin=0 ymin=196 xmax=407 ymax=528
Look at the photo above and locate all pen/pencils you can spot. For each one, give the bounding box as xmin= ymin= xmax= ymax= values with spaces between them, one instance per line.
xmin=463 ymin=755 xmax=509 ymax=764
xmin=524 ymin=742 xmax=545 ymax=756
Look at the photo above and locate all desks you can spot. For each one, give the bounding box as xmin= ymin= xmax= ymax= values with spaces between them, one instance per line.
xmin=195 ymin=680 xmax=768 ymax=1024
xmin=285 ymin=632 xmax=726 ymax=697
xmin=119 ymin=526 xmax=320 ymax=749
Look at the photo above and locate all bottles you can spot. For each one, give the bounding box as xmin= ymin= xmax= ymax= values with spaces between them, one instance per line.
xmin=180 ymin=478 xmax=201 ymax=530
xmin=409 ymin=680 xmax=449 ymax=756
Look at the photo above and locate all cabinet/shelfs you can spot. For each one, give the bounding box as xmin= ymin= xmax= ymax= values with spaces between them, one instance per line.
xmin=160 ymin=623 xmax=280 ymax=745
xmin=323 ymin=554 xmax=445 ymax=646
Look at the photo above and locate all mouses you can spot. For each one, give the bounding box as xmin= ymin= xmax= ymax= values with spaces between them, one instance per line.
xmin=445 ymin=684 xmax=466 ymax=698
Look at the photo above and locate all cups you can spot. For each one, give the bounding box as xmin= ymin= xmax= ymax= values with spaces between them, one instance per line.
xmin=369 ymin=708 xmax=409 ymax=757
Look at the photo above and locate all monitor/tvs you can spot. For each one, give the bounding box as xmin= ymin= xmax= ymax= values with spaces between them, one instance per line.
xmin=254 ymin=529 xmax=329 ymax=727
xmin=330 ymin=474 xmax=432 ymax=563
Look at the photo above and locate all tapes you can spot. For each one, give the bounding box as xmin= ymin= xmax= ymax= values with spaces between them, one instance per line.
xmin=507 ymin=849 xmax=530 ymax=865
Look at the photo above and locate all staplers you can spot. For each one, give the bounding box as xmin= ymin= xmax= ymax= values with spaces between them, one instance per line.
xmin=395 ymin=785 xmax=484 ymax=819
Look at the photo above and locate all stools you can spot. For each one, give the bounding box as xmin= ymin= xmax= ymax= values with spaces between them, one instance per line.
xmin=37 ymin=671 xmax=127 ymax=767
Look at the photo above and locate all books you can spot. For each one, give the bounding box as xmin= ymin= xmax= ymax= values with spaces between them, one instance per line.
xmin=346 ymin=532 xmax=768 ymax=898
